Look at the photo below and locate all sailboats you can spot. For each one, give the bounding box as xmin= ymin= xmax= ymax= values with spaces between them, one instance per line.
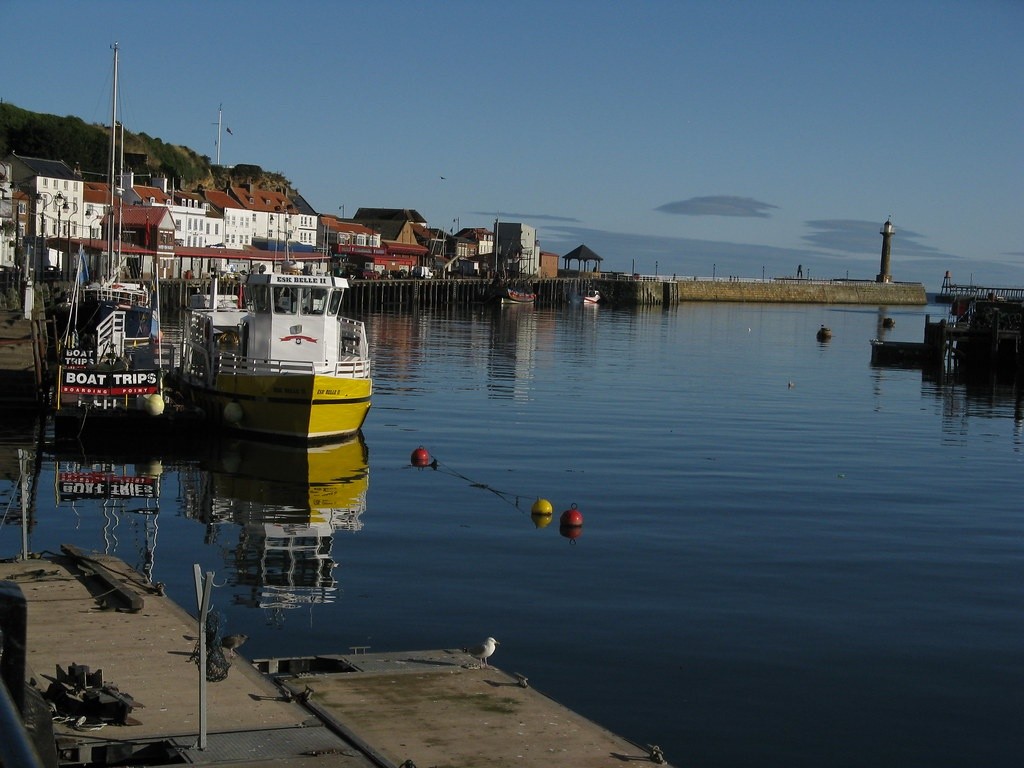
xmin=41 ymin=41 xmax=169 ymax=416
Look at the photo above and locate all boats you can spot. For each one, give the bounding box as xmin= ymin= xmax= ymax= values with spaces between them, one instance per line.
xmin=170 ymin=210 xmax=372 ymax=442
xmin=571 ymin=289 xmax=601 ymax=304
xmin=495 ymin=286 xmax=536 ymax=304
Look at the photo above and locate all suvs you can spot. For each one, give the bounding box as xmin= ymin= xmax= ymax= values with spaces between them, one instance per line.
xmin=359 ymin=269 xmax=380 ymax=280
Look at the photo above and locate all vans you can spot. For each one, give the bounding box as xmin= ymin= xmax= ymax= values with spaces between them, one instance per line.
xmin=413 ymin=267 xmax=433 ymax=279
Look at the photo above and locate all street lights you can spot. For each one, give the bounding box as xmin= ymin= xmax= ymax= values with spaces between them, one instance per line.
xmin=763 ymin=266 xmax=765 ymax=279
xmin=713 ymin=263 xmax=715 ymax=276
xmin=656 ymin=261 xmax=658 ymax=275
xmin=85 ymin=208 xmax=99 ymax=282
xmin=62 ymin=203 xmax=78 ymax=281
xmin=37 ymin=191 xmax=53 ymax=285
xmin=339 ymin=204 xmax=345 ymax=218
xmin=495 ymin=218 xmax=498 ymax=271
xmin=808 ymin=269 xmax=810 ymax=279
xmin=115 ymin=120 xmax=124 ymax=279
xmin=847 ymin=270 xmax=848 ymax=279
xmin=453 ymin=217 xmax=460 ymax=231
xmin=55 ymin=191 xmax=64 ymax=281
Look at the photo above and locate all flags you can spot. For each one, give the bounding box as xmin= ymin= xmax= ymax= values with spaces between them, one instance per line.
xmin=78 ymin=250 xmax=90 ymax=285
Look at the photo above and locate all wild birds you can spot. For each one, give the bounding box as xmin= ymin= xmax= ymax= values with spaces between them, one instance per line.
xmin=440 ymin=176 xmax=447 ymax=179
xmin=788 ymin=380 xmax=795 ymax=387
xmin=466 ymin=637 xmax=500 ymax=669
xmin=220 ymin=633 xmax=251 ymax=658
